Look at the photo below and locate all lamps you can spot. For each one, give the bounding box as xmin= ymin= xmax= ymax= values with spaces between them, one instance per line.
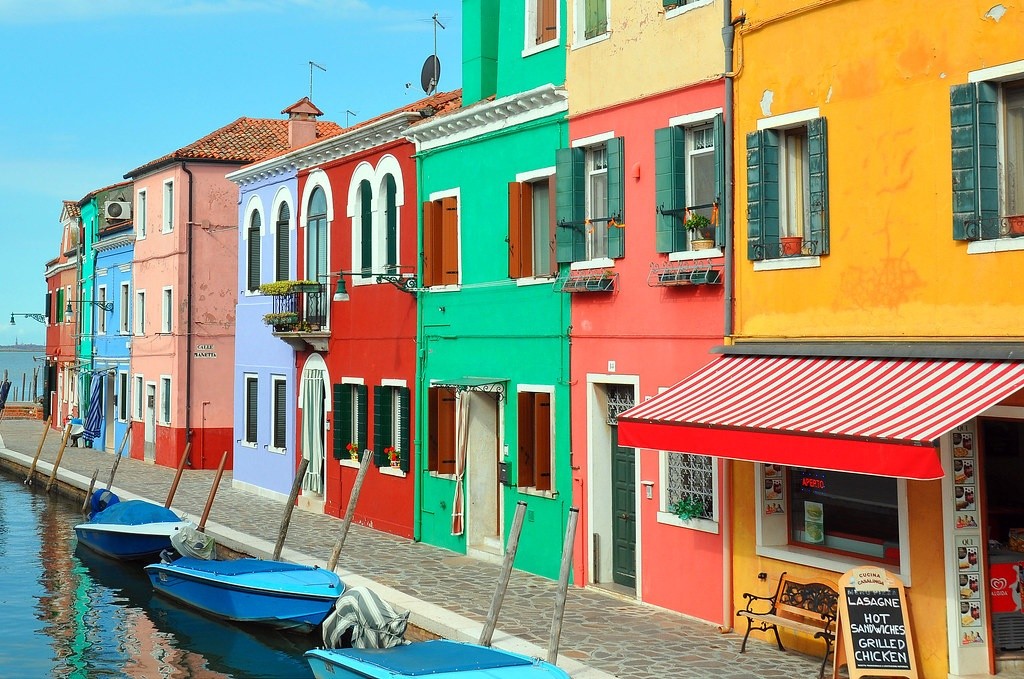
xmin=331 ymin=269 xmax=417 ymax=303
xmin=9 ymin=312 xmax=46 ymax=326
xmin=63 ymin=298 xmax=114 ymax=319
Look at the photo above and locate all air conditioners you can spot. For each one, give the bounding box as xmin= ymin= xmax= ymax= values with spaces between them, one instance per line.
xmin=104 ymin=201 xmax=131 ymax=219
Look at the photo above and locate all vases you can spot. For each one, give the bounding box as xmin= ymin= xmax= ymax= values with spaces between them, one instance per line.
xmin=350 ymin=453 xmax=358 ymax=461
xmin=1004 ymin=214 xmax=1024 ymax=238
xmin=389 ymin=461 xmax=400 ymax=470
xmin=780 ymin=237 xmax=803 ymax=255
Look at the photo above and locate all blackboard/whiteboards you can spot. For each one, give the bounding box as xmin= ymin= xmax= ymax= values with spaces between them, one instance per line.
xmin=832 ymin=565 xmax=918 ymax=679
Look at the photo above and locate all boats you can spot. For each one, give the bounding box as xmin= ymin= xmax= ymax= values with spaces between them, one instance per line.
xmin=143 ymin=525 xmax=346 ymax=634
xmin=72 ymin=487 xmax=198 ymax=560
xmin=302 ymin=586 xmax=572 ymax=679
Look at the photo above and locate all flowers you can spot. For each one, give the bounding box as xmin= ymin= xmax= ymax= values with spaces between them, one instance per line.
xmin=346 ymin=443 xmax=358 ymax=459
xmin=384 ymin=447 xmax=400 ymax=466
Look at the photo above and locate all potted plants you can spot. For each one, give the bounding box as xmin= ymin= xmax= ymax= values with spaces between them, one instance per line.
xmin=260 ymin=279 xmax=321 ymax=295
xmin=261 ymin=312 xmax=300 ymax=328
xmin=684 ymin=212 xmax=714 ymax=250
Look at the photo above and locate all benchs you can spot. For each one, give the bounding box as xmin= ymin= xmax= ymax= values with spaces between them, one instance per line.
xmin=739 ymin=571 xmax=841 ymax=679
